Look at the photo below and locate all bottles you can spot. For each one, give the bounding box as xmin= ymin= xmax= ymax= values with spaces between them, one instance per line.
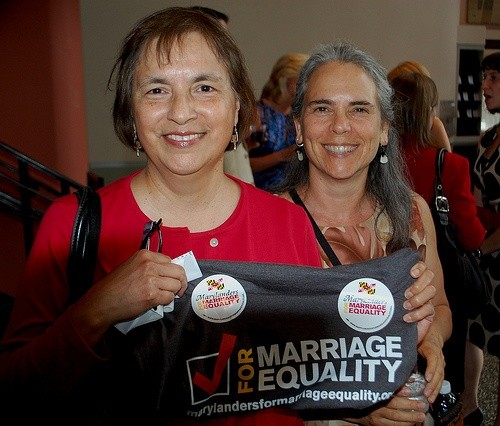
xmin=432 ymin=380 xmax=457 ymax=426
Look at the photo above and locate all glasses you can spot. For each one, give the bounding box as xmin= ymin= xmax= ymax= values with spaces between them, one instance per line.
xmin=141 ymin=217 xmax=163 ymax=253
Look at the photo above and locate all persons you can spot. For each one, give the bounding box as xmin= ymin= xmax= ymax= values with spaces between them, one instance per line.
xmin=188 ymin=7 xmax=500 ymax=426
xmin=0 ymin=7 xmax=437 ymax=426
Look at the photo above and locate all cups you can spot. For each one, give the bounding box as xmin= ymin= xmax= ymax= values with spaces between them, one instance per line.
xmin=250 ymin=124 xmax=268 ymax=143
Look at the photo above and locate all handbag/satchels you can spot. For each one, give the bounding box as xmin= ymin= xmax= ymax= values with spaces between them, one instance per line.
xmin=427 ymin=148 xmax=490 ymax=319
